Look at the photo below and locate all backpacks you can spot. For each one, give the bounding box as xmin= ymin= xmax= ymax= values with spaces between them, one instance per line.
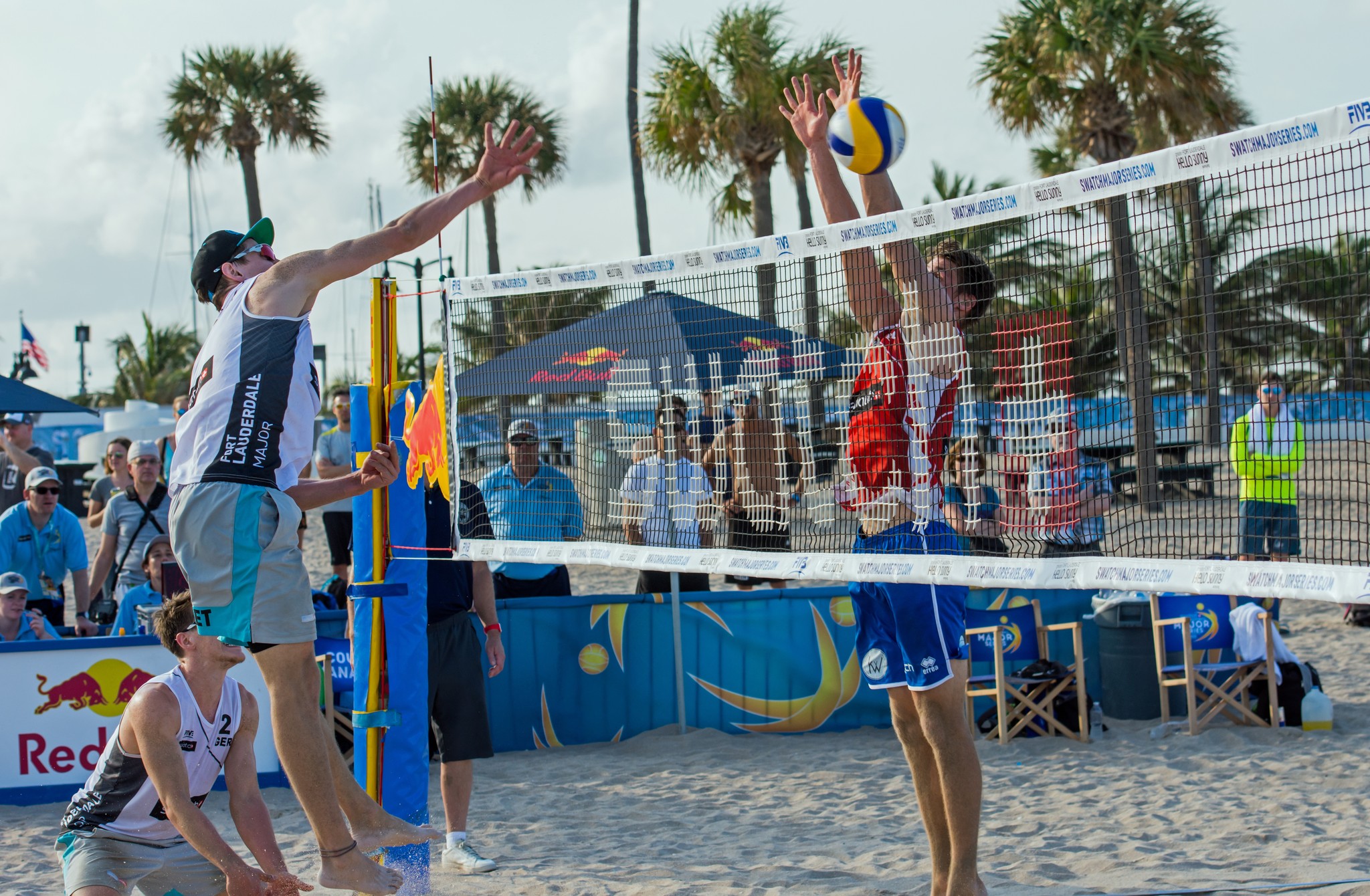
xmin=1010 ymin=658 xmax=1108 ymax=732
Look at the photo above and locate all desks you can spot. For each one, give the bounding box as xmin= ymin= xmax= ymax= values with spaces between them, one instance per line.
xmin=1011 ymin=439 xmax=1202 ymax=504
xmin=55 ymin=460 xmax=97 ymax=518
xmin=459 ymin=435 xmax=563 ymax=470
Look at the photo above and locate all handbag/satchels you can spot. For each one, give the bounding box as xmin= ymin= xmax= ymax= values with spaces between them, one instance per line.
xmin=1248 ymin=660 xmax=1324 ymax=726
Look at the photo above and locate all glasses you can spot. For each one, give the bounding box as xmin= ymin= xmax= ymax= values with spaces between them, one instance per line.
xmin=1051 ymin=428 xmax=1072 ymax=434
xmin=108 ymin=452 xmax=123 ymax=459
xmin=1262 ymin=387 xmax=1281 ymax=395
xmin=510 ymin=436 xmax=536 ymax=447
xmin=136 ymin=458 xmax=159 ymax=465
xmin=213 ymin=244 xmax=276 ymax=272
xmin=177 ymin=408 xmax=185 ymax=416
xmin=956 ymin=456 xmax=978 ymax=462
xmin=336 ymin=403 xmax=350 ymax=408
xmin=27 ymin=486 xmax=62 ymax=495
xmin=732 ymin=398 xmax=751 ymax=408
xmin=659 ymin=424 xmax=684 ymax=432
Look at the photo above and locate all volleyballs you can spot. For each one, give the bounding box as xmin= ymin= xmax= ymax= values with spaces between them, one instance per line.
xmin=826 ymin=97 xmax=906 ymax=175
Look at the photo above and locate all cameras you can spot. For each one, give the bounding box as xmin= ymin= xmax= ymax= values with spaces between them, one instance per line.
xmin=96 ymin=597 xmax=119 ymax=626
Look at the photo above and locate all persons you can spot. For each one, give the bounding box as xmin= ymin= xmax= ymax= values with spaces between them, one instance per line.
xmin=166 ymin=119 xmax=545 ymax=896
xmin=776 ymin=49 xmax=999 ymax=895
xmin=0 ymin=372 xmax=1306 ymax=896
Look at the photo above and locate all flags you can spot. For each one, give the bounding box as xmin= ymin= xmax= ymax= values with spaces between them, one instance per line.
xmin=20 ymin=323 xmax=50 ymax=371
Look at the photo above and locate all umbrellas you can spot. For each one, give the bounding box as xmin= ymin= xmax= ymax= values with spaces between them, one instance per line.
xmin=0 ymin=375 xmax=100 ymax=418
xmin=451 ymin=288 xmax=864 ymax=732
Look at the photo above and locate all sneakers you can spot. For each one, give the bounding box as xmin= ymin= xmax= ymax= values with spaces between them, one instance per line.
xmin=441 ymin=839 xmax=497 ymax=874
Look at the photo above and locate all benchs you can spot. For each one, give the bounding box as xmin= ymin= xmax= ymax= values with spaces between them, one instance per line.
xmin=460 ymin=450 xmax=574 ymax=466
xmin=993 ymin=470 xmax=1029 ymax=496
xmin=1110 ymin=463 xmax=1223 ymax=498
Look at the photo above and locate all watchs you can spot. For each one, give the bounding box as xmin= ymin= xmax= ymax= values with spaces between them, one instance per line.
xmin=483 ymin=622 xmax=501 ymax=635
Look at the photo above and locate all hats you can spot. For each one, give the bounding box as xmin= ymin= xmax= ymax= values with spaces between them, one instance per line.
xmin=1044 ymin=408 xmax=1076 ymax=430
xmin=190 ymin=218 xmax=275 ymax=294
xmin=733 ymin=384 xmax=755 ymax=400
xmin=0 ymin=412 xmax=33 ymax=425
xmin=127 ymin=440 xmax=160 ymax=462
xmin=508 ymin=420 xmax=536 ymax=439
xmin=25 ymin=465 xmax=63 ymax=489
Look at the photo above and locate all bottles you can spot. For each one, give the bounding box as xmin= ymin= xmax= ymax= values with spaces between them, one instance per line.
xmin=1089 ymin=701 xmax=1104 ymax=740
xmin=1148 ymin=721 xmax=1177 ymax=740
xmin=1301 ymin=686 xmax=1333 ymax=731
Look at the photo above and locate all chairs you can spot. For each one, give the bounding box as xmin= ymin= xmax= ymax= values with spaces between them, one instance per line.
xmin=1149 ymin=594 xmax=1279 ymax=735
xmin=963 ymin=599 xmax=1089 ymax=744
